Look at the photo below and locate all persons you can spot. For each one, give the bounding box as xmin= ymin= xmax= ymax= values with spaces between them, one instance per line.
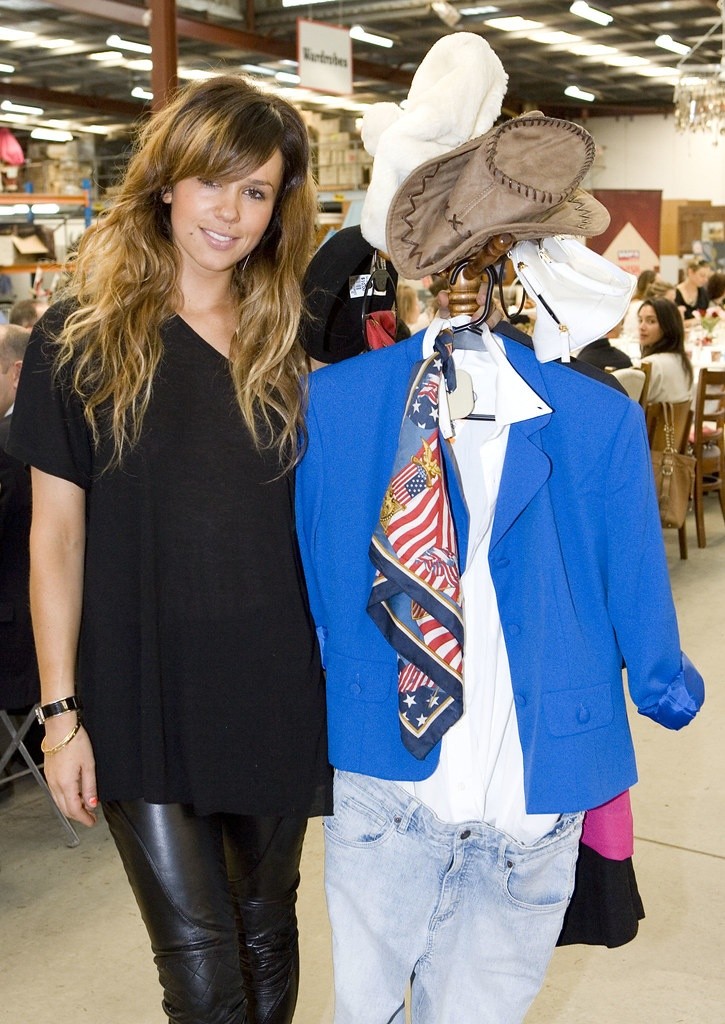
xmin=509 ymin=255 xmax=725 ymax=451
xmin=0 ymin=301 xmax=87 ymax=765
xmin=3 ymin=74 xmax=502 ymax=1024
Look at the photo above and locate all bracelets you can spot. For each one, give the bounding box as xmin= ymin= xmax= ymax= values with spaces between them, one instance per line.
xmin=35 ymin=694 xmax=79 ymax=725
xmin=40 ymin=722 xmax=82 ymax=756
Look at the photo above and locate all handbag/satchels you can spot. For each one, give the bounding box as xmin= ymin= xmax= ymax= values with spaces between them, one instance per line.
xmin=650 ymin=403 xmax=696 ymax=527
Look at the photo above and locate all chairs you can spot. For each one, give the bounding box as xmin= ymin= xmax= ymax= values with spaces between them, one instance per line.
xmin=604 ymin=362 xmax=725 ymax=561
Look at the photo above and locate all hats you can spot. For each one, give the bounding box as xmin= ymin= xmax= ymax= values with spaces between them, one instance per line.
xmin=507 ymin=228 xmax=637 ymax=363
xmin=295 ymin=224 xmax=398 ymax=365
xmin=386 ymin=114 xmax=609 ymax=279
